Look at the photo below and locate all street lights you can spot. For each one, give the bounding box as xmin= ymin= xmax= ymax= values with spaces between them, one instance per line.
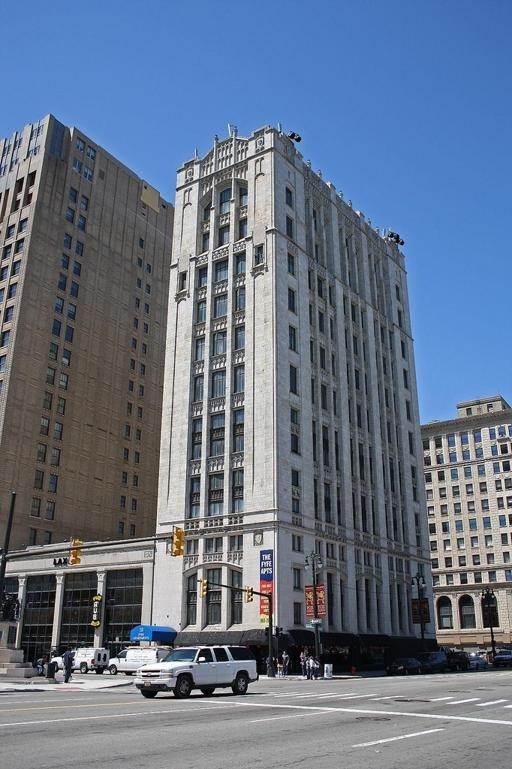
xmin=482 ymin=586 xmax=496 ymax=664
xmin=303 ymin=550 xmax=323 ymax=676
xmin=411 ymin=573 xmax=426 ymax=649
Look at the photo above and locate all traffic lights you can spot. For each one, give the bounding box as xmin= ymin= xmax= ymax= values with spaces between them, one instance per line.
xmin=244 ymin=586 xmax=254 ymax=603
xmin=199 ymin=579 xmax=210 ymax=599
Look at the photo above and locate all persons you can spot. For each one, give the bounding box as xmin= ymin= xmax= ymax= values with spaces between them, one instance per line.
xmin=260 ymin=649 xmax=293 ymax=679
xmin=36 ymin=654 xmax=47 ymax=677
xmin=300 ymin=647 xmax=321 ymax=680
xmin=63 ymin=648 xmax=74 ymax=683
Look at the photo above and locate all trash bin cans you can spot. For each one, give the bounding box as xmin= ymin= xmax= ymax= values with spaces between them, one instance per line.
xmin=47 ymin=662 xmax=55 ymax=678
xmin=323 ymin=664 xmax=333 ymax=679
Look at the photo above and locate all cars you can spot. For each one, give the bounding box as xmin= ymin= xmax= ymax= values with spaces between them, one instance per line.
xmin=494 ymin=650 xmax=511 ymax=668
xmin=108 ymin=646 xmax=171 ymax=675
xmin=385 ymin=651 xmax=486 ymax=674
xmin=51 ymin=647 xmax=109 ymax=674
xmin=134 ymin=646 xmax=260 ymax=698
